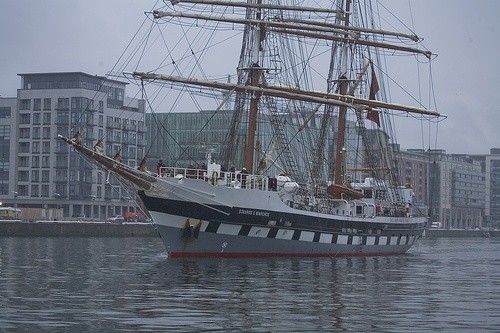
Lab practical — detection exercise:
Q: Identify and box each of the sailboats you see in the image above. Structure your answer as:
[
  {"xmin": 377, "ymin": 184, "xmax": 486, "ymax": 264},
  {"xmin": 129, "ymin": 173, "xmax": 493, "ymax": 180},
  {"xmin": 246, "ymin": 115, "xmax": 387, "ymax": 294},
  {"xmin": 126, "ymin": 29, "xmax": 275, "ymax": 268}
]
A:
[{"xmin": 56, "ymin": 0, "xmax": 448, "ymax": 260}]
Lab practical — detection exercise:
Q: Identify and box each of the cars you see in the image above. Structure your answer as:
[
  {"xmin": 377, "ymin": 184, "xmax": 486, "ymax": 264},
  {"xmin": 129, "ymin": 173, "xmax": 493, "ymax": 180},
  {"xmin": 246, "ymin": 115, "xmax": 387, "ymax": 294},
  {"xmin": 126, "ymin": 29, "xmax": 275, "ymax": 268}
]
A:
[{"xmin": 107, "ymin": 214, "xmax": 124, "ymax": 222}]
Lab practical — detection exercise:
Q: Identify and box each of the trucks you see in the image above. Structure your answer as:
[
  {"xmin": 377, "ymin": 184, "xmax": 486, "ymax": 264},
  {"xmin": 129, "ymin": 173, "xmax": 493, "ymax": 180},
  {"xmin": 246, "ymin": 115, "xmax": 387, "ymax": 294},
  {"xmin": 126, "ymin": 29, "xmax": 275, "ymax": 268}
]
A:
[{"xmin": 431, "ymin": 222, "xmax": 442, "ymax": 229}]
